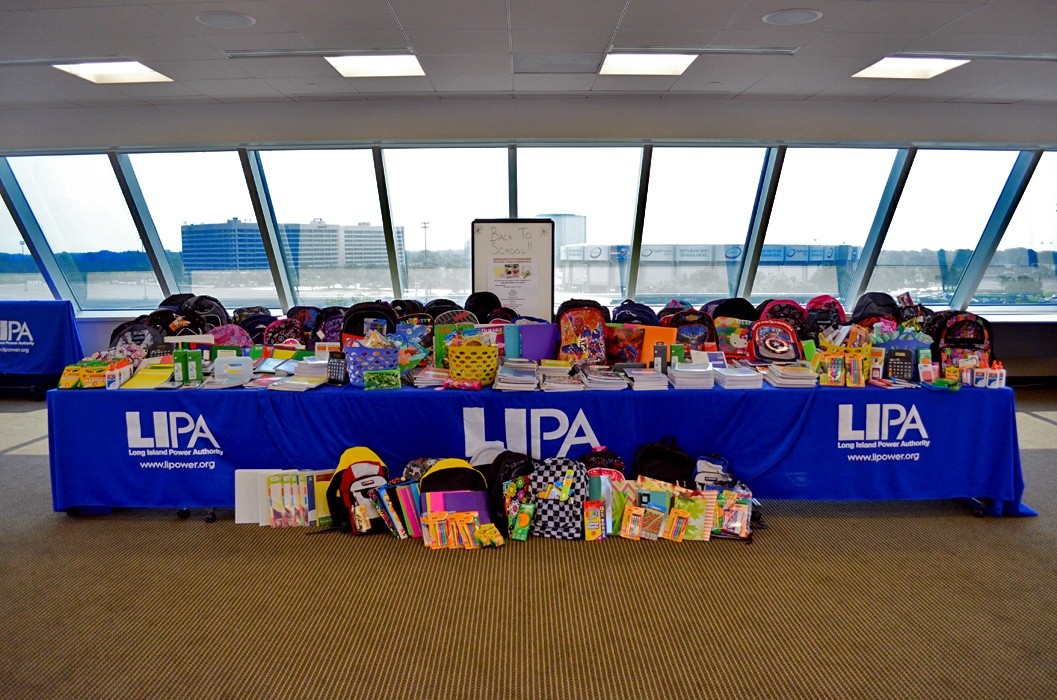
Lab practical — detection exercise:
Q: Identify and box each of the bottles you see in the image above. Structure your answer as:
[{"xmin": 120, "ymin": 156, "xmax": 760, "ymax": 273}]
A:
[
  {"xmin": 187, "ymin": 343, "xmax": 202, "ymax": 381},
  {"xmin": 998, "ymin": 361, "xmax": 1006, "ymax": 388},
  {"xmin": 174, "ymin": 343, "xmax": 190, "ymax": 383},
  {"xmin": 986, "ymin": 360, "xmax": 999, "ymax": 389},
  {"xmin": 106, "ymin": 358, "xmax": 134, "ymax": 389},
  {"xmin": 973, "ymin": 361, "xmax": 986, "ymax": 388},
  {"xmin": 984, "ymin": 360, "xmax": 990, "ymax": 387}
]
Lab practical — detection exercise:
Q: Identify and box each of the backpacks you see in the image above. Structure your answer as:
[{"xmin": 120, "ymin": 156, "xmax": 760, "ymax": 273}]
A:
[
  {"xmin": 659, "ymin": 299, "xmax": 719, "ymax": 360},
  {"xmin": 900, "ymin": 304, "xmax": 935, "ymax": 333},
  {"xmin": 755, "ymin": 300, "xmax": 807, "ymax": 343},
  {"xmin": 693, "ymin": 455, "xmax": 751, "ymax": 539},
  {"xmin": 578, "ymin": 447, "xmax": 624, "ymax": 474},
  {"xmin": 488, "ymin": 452, "xmax": 544, "ymax": 538},
  {"xmin": 555, "ymin": 298, "xmax": 611, "ymax": 365},
  {"xmin": 404, "ymin": 456, "xmax": 439, "ymax": 481},
  {"xmin": 749, "ymin": 320, "xmax": 804, "ymax": 369},
  {"xmin": 926, "ymin": 310, "xmax": 991, "ymax": 366},
  {"xmin": 612, "ymin": 298, "xmax": 659, "ymax": 325},
  {"xmin": 419, "ymin": 458, "xmax": 488, "ymax": 492},
  {"xmin": 109, "ymin": 292, "xmax": 544, "ymax": 360},
  {"xmin": 526, "ymin": 456, "xmax": 588, "ymax": 539},
  {"xmin": 632, "ymin": 437, "xmax": 695, "ymax": 489},
  {"xmin": 588, "ymin": 468, "xmax": 631, "ymax": 536},
  {"xmin": 804, "ymin": 294, "xmax": 848, "ymax": 347},
  {"xmin": 326, "ymin": 447, "xmax": 392, "ymax": 535},
  {"xmin": 851, "ymin": 292, "xmax": 900, "ymax": 331},
  {"xmin": 698, "ymin": 298, "xmax": 759, "ymax": 362}
]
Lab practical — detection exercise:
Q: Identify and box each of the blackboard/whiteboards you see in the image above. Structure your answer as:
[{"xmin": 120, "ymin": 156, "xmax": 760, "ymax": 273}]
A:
[{"xmin": 471, "ymin": 218, "xmax": 554, "ymax": 324}]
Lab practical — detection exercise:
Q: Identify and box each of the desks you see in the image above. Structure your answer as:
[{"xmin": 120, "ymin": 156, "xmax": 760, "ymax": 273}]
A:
[{"xmin": 47, "ymin": 383, "xmax": 1038, "ymax": 522}]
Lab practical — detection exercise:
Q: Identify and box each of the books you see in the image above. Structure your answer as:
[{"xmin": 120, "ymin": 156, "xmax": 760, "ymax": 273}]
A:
[
  {"xmin": 492, "ymin": 357, "xmax": 584, "ymax": 392},
  {"xmin": 434, "ymin": 325, "xmax": 557, "ymax": 367},
  {"xmin": 637, "ymin": 490, "xmax": 669, "ymax": 537},
  {"xmin": 121, "ymin": 357, "xmax": 326, "ymax": 391},
  {"xmin": 414, "ymin": 367, "xmax": 449, "ymax": 385},
  {"xmin": 615, "ymin": 363, "xmax": 668, "ymax": 390},
  {"xmin": 579, "ymin": 370, "xmax": 629, "ymax": 390},
  {"xmin": 667, "ymin": 362, "xmax": 714, "ymax": 389},
  {"xmin": 368, "ymin": 480, "xmax": 487, "ymax": 547},
  {"xmin": 733, "ymin": 358, "xmax": 818, "ymax": 387},
  {"xmin": 713, "ymin": 367, "xmax": 763, "ymax": 388}
]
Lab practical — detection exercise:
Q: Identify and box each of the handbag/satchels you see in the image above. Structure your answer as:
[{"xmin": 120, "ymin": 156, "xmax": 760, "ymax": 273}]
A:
[
  {"xmin": 344, "ymin": 337, "xmax": 401, "ymax": 386},
  {"xmin": 448, "ymin": 338, "xmax": 500, "ymax": 386},
  {"xmin": 872, "ymin": 332, "xmax": 931, "ymax": 384}
]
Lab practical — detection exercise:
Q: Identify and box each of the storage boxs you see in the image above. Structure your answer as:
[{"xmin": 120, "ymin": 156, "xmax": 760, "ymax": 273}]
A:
[
  {"xmin": 215, "ymin": 356, "xmax": 254, "ymax": 380},
  {"xmin": 605, "ymin": 324, "xmax": 677, "ymax": 367}
]
